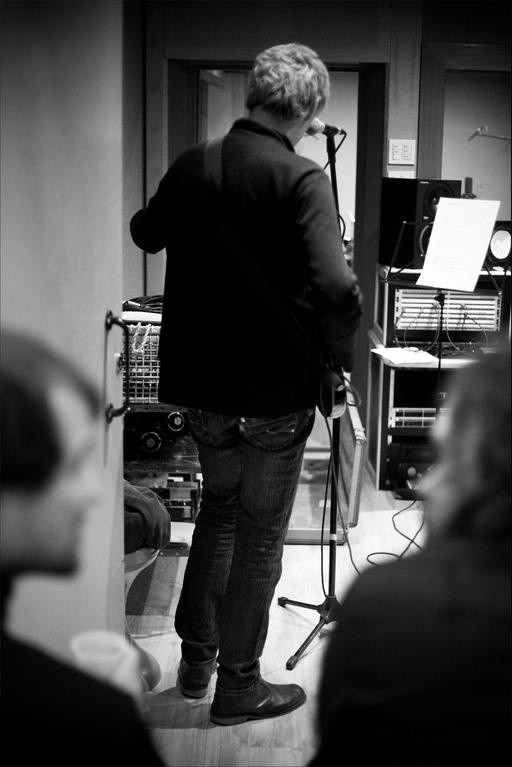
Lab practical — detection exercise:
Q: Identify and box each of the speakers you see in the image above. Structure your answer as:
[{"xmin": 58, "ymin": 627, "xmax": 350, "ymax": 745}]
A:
[{"xmin": 378, "ymin": 176, "xmax": 462, "ymax": 269}]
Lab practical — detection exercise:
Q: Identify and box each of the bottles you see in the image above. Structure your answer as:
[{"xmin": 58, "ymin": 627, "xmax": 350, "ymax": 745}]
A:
[{"xmin": 462, "ymin": 175, "xmax": 475, "ymax": 198}]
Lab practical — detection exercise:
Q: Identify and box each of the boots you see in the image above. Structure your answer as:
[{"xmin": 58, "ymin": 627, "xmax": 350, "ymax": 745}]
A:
[
  {"xmin": 176, "ymin": 640, "xmax": 218, "ymax": 700},
  {"xmin": 210, "ymin": 663, "xmax": 306, "ymax": 726}
]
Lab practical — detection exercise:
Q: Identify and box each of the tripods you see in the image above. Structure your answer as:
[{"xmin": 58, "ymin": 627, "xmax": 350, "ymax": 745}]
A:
[{"xmin": 278, "ymin": 139, "xmax": 344, "ymax": 670}]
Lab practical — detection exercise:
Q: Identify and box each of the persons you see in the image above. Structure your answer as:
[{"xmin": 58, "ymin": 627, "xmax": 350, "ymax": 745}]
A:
[
  {"xmin": 296, "ymin": 345, "xmax": 509, "ymax": 764},
  {"xmin": 0, "ymin": 331, "xmax": 164, "ymax": 767},
  {"xmin": 128, "ymin": 41, "xmax": 367, "ymax": 727}
]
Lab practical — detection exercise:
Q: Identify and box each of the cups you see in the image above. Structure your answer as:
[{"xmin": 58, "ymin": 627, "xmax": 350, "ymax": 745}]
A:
[{"xmin": 69, "ymin": 630, "xmax": 130, "ymax": 680}]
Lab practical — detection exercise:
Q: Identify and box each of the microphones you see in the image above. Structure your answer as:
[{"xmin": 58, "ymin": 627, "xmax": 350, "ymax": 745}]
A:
[
  {"xmin": 305, "ymin": 117, "xmax": 345, "ymax": 135},
  {"xmin": 467, "ymin": 126, "xmax": 485, "ymax": 142}
]
